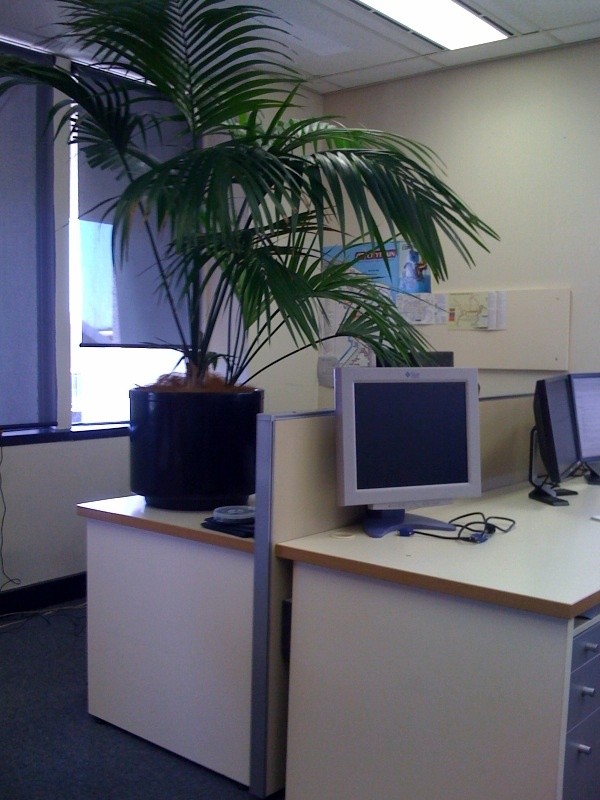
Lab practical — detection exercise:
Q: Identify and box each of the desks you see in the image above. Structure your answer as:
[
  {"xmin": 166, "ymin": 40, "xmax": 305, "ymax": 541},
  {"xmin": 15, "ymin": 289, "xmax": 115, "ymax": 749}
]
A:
[
  {"xmin": 75, "ymin": 493, "xmax": 255, "ymax": 787},
  {"xmin": 275, "ymin": 470, "xmax": 600, "ymax": 800}
]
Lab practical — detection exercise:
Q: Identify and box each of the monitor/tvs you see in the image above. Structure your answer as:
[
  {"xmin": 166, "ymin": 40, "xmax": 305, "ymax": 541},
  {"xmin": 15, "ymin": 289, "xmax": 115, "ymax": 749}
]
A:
[
  {"xmin": 334, "ymin": 365, "xmax": 484, "ymax": 538},
  {"xmin": 526, "ymin": 372, "xmax": 600, "ymax": 507}
]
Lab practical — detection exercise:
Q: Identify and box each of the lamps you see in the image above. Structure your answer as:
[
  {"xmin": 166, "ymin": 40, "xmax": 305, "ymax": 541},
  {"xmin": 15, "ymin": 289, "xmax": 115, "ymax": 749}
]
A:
[{"xmin": 316, "ymin": 334, "xmax": 371, "ymax": 389}]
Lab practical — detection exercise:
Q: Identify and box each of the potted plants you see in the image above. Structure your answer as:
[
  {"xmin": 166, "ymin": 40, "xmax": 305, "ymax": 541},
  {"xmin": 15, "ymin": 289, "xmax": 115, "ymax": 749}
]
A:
[{"xmin": 0, "ymin": 0, "xmax": 498, "ymax": 530}]
